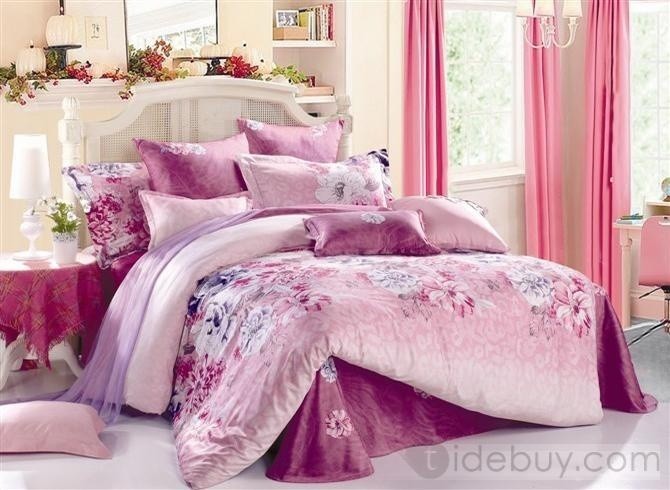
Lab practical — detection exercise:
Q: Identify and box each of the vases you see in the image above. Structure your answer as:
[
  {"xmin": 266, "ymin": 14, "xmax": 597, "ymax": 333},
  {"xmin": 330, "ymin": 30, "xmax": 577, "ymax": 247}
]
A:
[{"xmin": 52, "ymin": 232, "xmax": 80, "ymax": 265}]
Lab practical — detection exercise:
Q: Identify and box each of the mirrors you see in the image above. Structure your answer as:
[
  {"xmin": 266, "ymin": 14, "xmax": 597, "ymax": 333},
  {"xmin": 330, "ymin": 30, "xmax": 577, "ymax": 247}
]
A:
[{"xmin": 125, "ymin": 0, "xmax": 218, "ymax": 69}]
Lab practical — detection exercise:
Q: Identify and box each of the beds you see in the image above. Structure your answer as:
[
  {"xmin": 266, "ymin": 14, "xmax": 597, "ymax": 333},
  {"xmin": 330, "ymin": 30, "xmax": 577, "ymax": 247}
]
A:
[{"xmin": 56, "ymin": 74, "xmax": 659, "ymax": 489}]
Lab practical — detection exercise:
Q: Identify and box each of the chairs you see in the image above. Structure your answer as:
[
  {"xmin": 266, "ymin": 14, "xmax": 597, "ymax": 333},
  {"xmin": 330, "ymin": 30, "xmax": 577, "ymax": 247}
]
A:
[{"xmin": 625, "ymin": 215, "xmax": 669, "ymax": 351}]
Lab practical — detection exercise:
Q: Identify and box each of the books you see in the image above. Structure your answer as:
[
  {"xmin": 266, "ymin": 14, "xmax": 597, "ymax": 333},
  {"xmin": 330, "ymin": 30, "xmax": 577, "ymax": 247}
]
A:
[{"xmin": 299, "ymin": 4, "xmax": 328, "ymax": 40}]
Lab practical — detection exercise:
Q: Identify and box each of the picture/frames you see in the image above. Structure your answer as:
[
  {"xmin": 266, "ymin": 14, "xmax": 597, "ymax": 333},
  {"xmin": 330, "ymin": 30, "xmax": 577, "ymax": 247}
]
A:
[
  {"xmin": 58, "ymin": 0, "xmax": 129, "ymax": 76},
  {"xmin": 275, "ymin": 9, "xmax": 300, "ymax": 28}
]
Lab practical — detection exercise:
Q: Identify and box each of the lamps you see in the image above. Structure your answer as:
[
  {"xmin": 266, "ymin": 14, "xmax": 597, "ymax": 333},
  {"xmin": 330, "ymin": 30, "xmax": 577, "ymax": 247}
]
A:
[
  {"xmin": 8, "ymin": 133, "xmax": 55, "ymax": 262},
  {"xmin": 44, "ymin": 11, "xmax": 85, "ymax": 70},
  {"xmin": 14, "ymin": 38, "xmax": 47, "ymax": 80},
  {"xmin": 513, "ymin": 0, "xmax": 583, "ymax": 51},
  {"xmin": 171, "ymin": 40, "xmax": 278, "ymax": 77}
]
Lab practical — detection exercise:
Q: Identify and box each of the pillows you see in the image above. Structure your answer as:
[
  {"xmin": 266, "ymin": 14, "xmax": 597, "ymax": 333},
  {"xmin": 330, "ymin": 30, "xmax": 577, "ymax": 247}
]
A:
[
  {"xmin": 0, "ymin": 400, "xmax": 116, "ymax": 464},
  {"xmin": 62, "ymin": 112, "xmax": 513, "ymax": 261}
]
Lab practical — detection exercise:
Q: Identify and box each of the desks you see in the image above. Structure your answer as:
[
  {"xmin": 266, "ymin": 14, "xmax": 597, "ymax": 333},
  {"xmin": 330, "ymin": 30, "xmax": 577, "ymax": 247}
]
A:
[
  {"xmin": 612, "ymin": 200, "xmax": 670, "ymax": 330},
  {"xmin": 0, "ymin": 252, "xmax": 102, "ymax": 395}
]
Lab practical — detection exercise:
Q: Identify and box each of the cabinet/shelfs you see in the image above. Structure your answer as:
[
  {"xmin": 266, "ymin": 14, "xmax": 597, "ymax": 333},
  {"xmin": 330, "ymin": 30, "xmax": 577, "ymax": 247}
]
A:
[{"xmin": 271, "ymin": 40, "xmax": 337, "ymax": 104}]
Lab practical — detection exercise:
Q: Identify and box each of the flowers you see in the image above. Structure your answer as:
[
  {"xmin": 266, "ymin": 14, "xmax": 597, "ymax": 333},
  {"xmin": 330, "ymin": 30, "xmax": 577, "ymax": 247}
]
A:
[{"xmin": 22, "ymin": 193, "xmax": 83, "ymax": 242}]
